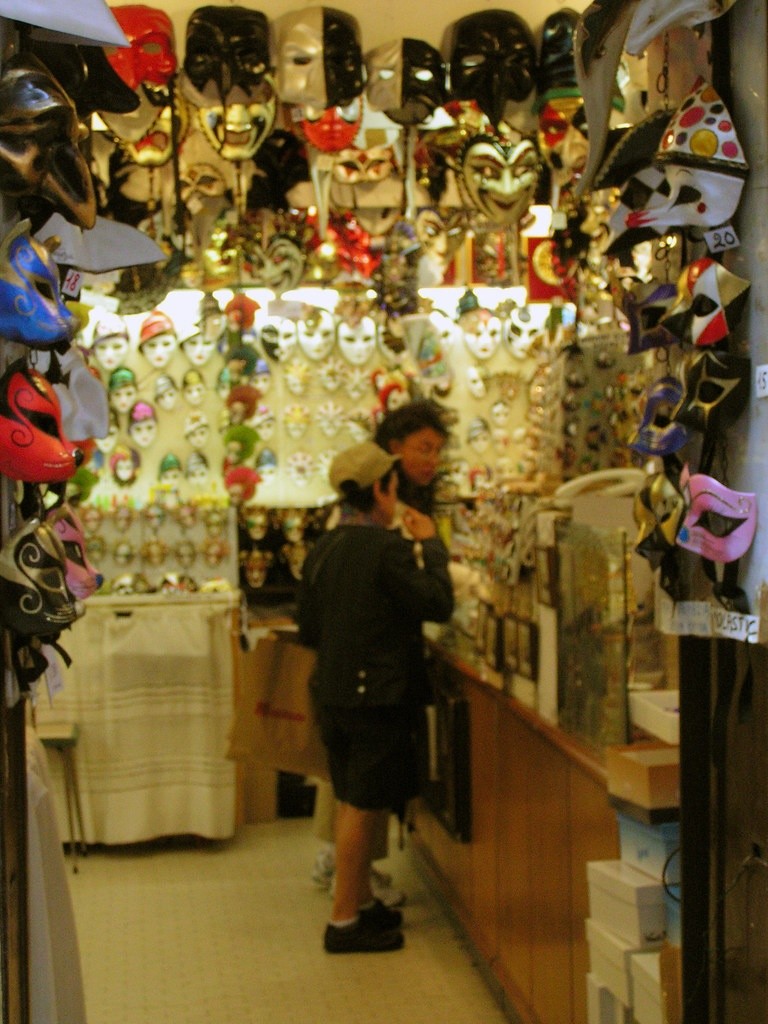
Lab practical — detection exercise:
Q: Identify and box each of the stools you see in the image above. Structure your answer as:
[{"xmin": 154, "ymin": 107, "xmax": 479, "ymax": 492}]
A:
[{"xmin": 37, "ymin": 721, "xmax": 91, "ymax": 874}]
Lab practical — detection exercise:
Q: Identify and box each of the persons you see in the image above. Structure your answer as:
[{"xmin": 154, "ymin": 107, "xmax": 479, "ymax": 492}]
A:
[
  {"xmin": 311, "ymin": 403, "xmax": 447, "ymax": 905},
  {"xmin": 297, "ymin": 441, "xmax": 458, "ymax": 953}
]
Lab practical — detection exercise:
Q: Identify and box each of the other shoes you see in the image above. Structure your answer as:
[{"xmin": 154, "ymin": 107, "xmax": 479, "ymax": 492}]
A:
[
  {"xmin": 332, "ymin": 899, "xmax": 407, "ymax": 928},
  {"xmin": 325, "ymin": 918, "xmax": 406, "ymax": 954},
  {"xmin": 328, "ymin": 874, "xmax": 409, "ymax": 906},
  {"xmin": 308, "ymin": 848, "xmax": 393, "ymax": 889}
]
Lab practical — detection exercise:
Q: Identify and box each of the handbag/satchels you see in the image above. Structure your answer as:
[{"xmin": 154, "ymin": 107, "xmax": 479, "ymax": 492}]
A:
[{"xmin": 225, "ymin": 630, "xmax": 335, "ymax": 786}]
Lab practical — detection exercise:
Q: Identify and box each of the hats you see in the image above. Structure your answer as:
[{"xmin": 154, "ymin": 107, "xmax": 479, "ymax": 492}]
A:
[{"xmin": 329, "ymin": 441, "xmax": 401, "ymax": 494}]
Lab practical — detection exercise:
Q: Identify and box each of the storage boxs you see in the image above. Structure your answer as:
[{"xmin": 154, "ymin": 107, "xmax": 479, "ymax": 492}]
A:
[{"xmin": 583, "ymin": 688, "xmax": 680, "ymax": 1022}]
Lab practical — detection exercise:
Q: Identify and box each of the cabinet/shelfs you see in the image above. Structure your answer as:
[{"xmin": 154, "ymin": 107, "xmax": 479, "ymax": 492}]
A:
[{"xmin": 415, "ymin": 675, "xmax": 672, "ymax": 1024}]
